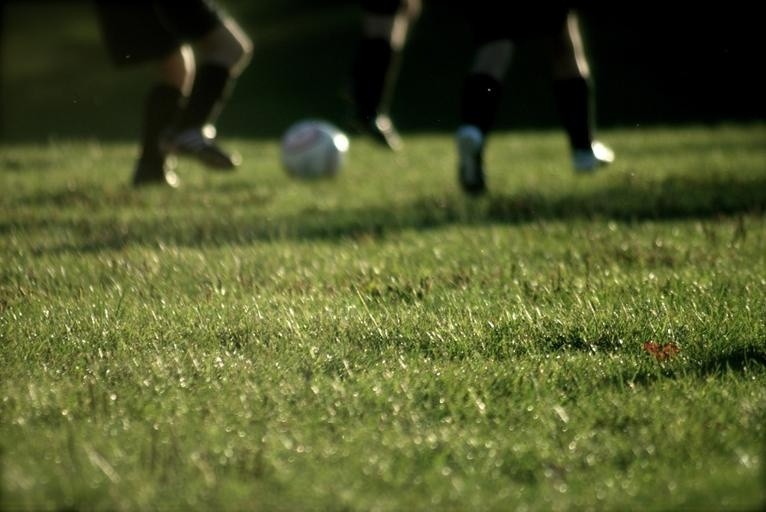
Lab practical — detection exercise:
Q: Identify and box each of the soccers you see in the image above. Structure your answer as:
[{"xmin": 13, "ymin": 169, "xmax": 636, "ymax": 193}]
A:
[{"xmin": 283, "ymin": 123, "xmax": 345, "ymax": 181}]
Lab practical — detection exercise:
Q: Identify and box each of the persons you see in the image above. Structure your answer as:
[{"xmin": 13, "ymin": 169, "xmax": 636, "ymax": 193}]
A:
[
  {"xmin": 353, "ymin": 1, "xmax": 423, "ymax": 151},
  {"xmin": 452, "ymin": 3, "xmax": 617, "ymax": 196},
  {"xmin": 91, "ymin": 0, "xmax": 253, "ymax": 190}
]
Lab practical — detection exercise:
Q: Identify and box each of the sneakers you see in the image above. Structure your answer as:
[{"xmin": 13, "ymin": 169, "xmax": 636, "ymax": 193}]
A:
[
  {"xmin": 457, "ymin": 126, "xmax": 488, "ymax": 197},
  {"xmin": 156, "ymin": 122, "xmax": 239, "ymax": 170},
  {"xmin": 136, "ymin": 152, "xmax": 179, "ymax": 188},
  {"xmin": 573, "ymin": 142, "xmax": 616, "ymax": 169}
]
